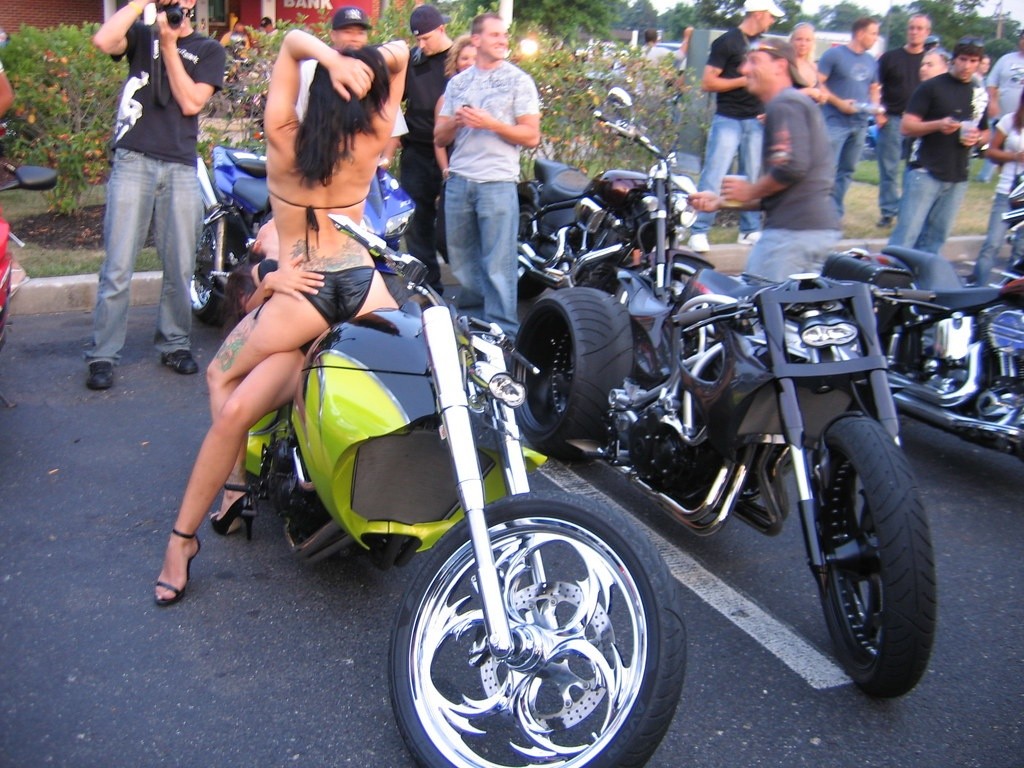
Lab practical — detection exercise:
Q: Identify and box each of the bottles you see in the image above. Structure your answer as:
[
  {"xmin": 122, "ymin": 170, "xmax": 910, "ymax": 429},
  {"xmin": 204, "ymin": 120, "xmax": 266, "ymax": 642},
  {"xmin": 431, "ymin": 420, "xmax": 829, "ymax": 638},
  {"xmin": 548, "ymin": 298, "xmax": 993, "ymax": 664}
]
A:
[{"xmin": 853, "ymin": 102, "xmax": 884, "ymax": 114}]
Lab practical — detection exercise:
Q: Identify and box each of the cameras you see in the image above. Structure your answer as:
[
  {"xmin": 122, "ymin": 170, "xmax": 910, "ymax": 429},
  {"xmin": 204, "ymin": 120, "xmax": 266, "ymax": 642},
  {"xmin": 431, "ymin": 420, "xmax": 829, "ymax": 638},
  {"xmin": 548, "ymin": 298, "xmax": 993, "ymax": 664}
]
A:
[{"xmin": 156, "ymin": 0, "xmax": 186, "ymax": 29}]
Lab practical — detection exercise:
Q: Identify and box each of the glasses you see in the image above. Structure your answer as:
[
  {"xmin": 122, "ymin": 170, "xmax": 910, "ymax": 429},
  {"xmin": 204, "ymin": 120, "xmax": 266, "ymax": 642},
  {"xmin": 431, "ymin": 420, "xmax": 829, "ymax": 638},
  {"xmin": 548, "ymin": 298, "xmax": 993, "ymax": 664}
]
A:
[
  {"xmin": 747, "ymin": 43, "xmax": 778, "ymax": 51},
  {"xmin": 957, "ymin": 38, "xmax": 985, "ymax": 47}
]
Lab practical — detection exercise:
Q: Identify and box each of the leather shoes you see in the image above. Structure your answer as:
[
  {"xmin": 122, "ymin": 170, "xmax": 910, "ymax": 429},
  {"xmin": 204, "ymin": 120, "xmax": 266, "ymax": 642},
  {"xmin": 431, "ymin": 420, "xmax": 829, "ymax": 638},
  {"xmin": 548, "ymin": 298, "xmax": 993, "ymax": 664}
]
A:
[
  {"xmin": 162, "ymin": 349, "xmax": 199, "ymax": 374},
  {"xmin": 87, "ymin": 361, "xmax": 113, "ymax": 389}
]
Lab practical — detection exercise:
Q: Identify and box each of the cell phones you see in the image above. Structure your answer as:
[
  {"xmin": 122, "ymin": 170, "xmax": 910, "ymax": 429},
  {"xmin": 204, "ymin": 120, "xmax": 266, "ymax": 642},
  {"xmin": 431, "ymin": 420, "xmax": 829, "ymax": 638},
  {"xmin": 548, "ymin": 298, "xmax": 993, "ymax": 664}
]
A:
[{"xmin": 462, "ymin": 104, "xmax": 470, "ymax": 108}]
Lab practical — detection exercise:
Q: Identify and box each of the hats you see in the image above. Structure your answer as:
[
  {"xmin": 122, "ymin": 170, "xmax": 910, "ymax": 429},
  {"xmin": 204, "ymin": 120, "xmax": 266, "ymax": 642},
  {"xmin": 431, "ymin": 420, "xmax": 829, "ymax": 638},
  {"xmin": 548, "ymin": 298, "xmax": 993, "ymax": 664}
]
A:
[
  {"xmin": 410, "ymin": 5, "xmax": 452, "ymax": 36},
  {"xmin": 332, "ymin": 7, "xmax": 373, "ymax": 29},
  {"xmin": 743, "ymin": 0, "xmax": 786, "ymax": 18},
  {"xmin": 749, "ymin": 36, "xmax": 808, "ymax": 89},
  {"xmin": 260, "ymin": 17, "xmax": 271, "ymax": 27}
]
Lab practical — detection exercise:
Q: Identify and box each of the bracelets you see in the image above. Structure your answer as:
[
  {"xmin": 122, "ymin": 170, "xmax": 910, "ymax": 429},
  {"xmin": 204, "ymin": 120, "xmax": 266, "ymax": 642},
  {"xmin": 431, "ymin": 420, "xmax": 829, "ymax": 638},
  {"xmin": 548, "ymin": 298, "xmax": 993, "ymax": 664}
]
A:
[
  {"xmin": 977, "ymin": 134, "xmax": 983, "ymax": 143},
  {"xmin": 129, "ymin": 1, "xmax": 142, "ymax": 15}
]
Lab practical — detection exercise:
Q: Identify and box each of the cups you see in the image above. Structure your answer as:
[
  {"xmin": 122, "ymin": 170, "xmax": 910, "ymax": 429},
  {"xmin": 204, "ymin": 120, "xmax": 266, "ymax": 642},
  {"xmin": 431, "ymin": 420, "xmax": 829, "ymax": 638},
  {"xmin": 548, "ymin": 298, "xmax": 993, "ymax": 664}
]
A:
[
  {"xmin": 959, "ymin": 121, "xmax": 976, "ymax": 141},
  {"xmin": 722, "ymin": 175, "xmax": 747, "ymax": 209}
]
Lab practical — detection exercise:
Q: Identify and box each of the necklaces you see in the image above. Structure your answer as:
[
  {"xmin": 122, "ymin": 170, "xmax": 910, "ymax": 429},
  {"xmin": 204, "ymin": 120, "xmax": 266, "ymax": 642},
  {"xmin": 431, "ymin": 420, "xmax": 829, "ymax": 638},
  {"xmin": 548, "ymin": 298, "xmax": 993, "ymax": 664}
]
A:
[{"xmin": 741, "ymin": 29, "xmax": 759, "ymax": 46}]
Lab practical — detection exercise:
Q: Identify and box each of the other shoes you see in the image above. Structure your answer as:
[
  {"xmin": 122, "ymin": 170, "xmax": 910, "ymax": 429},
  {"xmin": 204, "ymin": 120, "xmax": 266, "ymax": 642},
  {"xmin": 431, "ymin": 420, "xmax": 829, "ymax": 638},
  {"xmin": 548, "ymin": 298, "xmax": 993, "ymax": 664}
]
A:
[{"xmin": 876, "ymin": 216, "xmax": 892, "ymax": 228}]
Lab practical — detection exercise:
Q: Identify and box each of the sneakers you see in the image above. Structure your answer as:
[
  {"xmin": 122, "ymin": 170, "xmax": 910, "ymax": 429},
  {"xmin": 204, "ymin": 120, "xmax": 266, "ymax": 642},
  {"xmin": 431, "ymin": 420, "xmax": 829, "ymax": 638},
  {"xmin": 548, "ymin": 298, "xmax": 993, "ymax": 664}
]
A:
[
  {"xmin": 687, "ymin": 233, "xmax": 710, "ymax": 251},
  {"xmin": 737, "ymin": 231, "xmax": 761, "ymax": 244}
]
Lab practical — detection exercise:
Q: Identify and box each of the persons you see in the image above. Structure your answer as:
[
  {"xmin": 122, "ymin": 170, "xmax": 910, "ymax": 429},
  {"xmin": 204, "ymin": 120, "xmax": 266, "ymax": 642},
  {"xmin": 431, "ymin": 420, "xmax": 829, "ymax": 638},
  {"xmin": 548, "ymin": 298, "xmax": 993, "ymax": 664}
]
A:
[
  {"xmin": 645, "ymin": 1, "xmax": 1024, "ymax": 286},
  {"xmin": 295, "ymin": 7, "xmax": 408, "ymax": 171},
  {"xmin": 0, "ymin": 61, "xmax": 13, "ymax": 118},
  {"xmin": 207, "ymin": 30, "xmax": 409, "ymax": 542},
  {"xmin": 220, "ymin": 21, "xmax": 250, "ymax": 50},
  {"xmin": 433, "ymin": 14, "xmax": 541, "ymax": 372},
  {"xmin": 85, "ymin": 0, "xmax": 225, "ymax": 389},
  {"xmin": 402, "ymin": 6, "xmax": 478, "ymax": 297},
  {"xmin": 154, "ymin": 217, "xmax": 321, "ymax": 605},
  {"xmin": 258, "ymin": 17, "xmax": 278, "ymax": 37}
]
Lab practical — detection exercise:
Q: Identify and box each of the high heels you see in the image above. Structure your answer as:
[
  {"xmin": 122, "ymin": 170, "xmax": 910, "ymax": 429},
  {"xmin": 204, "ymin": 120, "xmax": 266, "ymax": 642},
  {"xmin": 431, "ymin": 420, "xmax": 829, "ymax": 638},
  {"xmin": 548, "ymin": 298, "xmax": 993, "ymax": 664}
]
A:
[
  {"xmin": 211, "ymin": 484, "xmax": 259, "ymax": 541},
  {"xmin": 154, "ymin": 527, "xmax": 200, "ymax": 606}
]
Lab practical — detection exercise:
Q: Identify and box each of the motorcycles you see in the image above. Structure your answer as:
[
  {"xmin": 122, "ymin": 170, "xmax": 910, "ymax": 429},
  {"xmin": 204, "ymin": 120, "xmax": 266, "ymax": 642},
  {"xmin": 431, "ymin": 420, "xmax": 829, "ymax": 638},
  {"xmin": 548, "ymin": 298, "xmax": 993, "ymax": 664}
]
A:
[
  {"xmin": 0, "ymin": 166, "xmax": 59, "ymax": 408},
  {"xmin": 519, "ymin": 88, "xmax": 716, "ymax": 384},
  {"xmin": 509, "ymin": 255, "xmax": 936, "ymax": 696},
  {"xmin": 244, "ymin": 213, "xmax": 688, "ymax": 768},
  {"xmin": 790, "ymin": 168, "xmax": 1024, "ymax": 458},
  {"xmin": 189, "ymin": 140, "xmax": 417, "ymax": 324}
]
